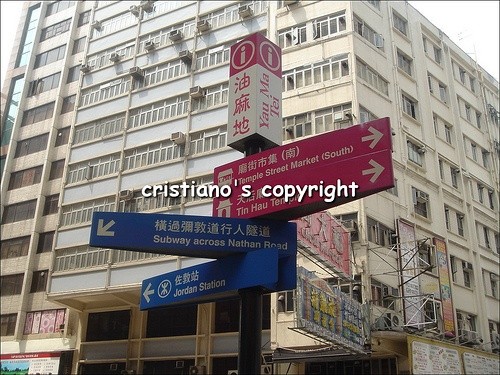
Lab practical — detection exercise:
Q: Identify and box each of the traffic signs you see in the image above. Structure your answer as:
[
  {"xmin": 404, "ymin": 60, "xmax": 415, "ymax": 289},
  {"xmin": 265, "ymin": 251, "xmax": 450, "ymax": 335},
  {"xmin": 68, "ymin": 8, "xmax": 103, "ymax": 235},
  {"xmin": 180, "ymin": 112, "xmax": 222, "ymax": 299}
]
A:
[
  {"xmin": 141, "ymin": 259, "xmax": 297, "ymax": 311},
  {"xmin": 89, "ymin": 211, "xmax": 298, "ymax": 259},
  {"xmin": 212, "ymin": 149, "xmax": 393, "ymax": 222},
  {"xmin": 213, "ymin": 117, "xmax": 393, "ymax": 197}
]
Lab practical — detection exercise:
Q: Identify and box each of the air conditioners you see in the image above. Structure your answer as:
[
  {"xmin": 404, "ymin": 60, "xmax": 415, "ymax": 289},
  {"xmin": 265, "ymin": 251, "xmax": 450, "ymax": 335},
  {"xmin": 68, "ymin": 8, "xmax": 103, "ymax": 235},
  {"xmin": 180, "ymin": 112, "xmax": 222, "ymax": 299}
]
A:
[
  {"xmin": 171, "ymin": 131, "xmax": 185, "ymax": 144},
  {"xmin": 119, "ymin": 189, "xmax": 132, "ymax": 200},
  {"xmin": 109, "ymin": 53, "xmax": 120, "ymax": 61},
  {"xmin": 196, "ymin": 19, "xmax": 211, "ymax": 31},
  {"xmin": 178, "ymin": 49, "xmax": 192, "ymax": 63},
  {"xmin": 169, "ymin": 30, "xmax": 183, "ymax": 40},
  {"xmin": 384, "ymin": 313, "xmax": 402, "ymax": 332},
  {"xmin": 129, "ymin": 5, "xmax": 138, "ymax": 15},
  {"xmin": 140, "ymin": 0, "xmax": 152, "ymax": 9},
  {"xmin": 145, "ymin": 41, "xmax": 154, "ymax": 50},
  {"xmin": 129, "ymin": 66, "xmax": 143, "ymax": 79},
  {"xmin": 238, "ymin": 5, "xmax": 252, "ymax": 19},
  {"xmin": 91, "ymin": 19, "xmax": 99, "ymax": 28},
  {"xmin": 80, "ymin": 64, "xmax": 90, "ymax": 72},
  {"xmin": 190, "ymin": 85, "xmax": 203, "ymax": 98}
]
